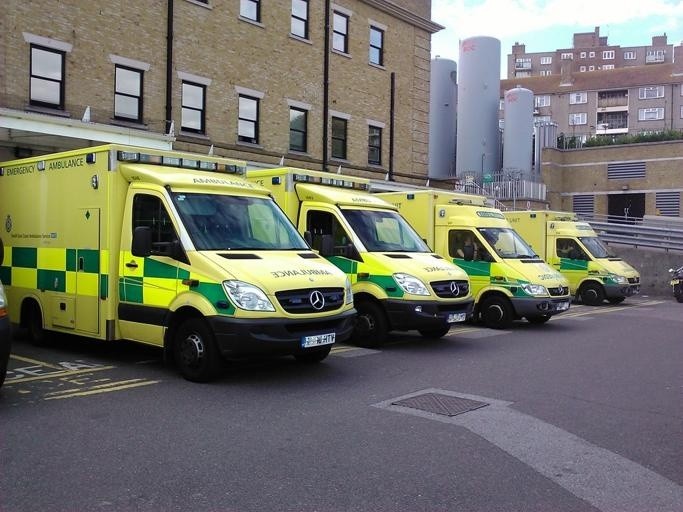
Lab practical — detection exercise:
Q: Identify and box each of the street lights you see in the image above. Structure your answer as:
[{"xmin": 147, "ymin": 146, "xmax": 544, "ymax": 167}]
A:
[{"xmin": 480, "ymin": 153, "xmax": 487, "ymax": 180}]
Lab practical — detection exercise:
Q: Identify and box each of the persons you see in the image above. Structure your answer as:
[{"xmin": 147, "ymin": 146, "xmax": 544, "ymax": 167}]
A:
[
  {"xmin": 478, "ymin": 232, "xmax": 499, "ymax": 260},
  {"xmin": 455, "ymin": 235, "xmax": 478, "ymax": 260}
]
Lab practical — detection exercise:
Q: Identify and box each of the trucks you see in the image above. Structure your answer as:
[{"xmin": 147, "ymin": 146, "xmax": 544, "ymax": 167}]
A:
[
  {"xmin": 0, "ymin": 237, "xmax": 10, "ymax": 380},
  {"xmin": 500, "ymin": 209, "xmax": 641, "ymax": 306},
  {"xmin": 244, "ymin": 167, "xmax": 477, "ymax": 347},
  {"xmin": 369, "ymin": 187, "xmax": 574, "ymax": 333},
  {"xmin": 0, "ymin": 144, "xmax": 358, "ymax": 385}
]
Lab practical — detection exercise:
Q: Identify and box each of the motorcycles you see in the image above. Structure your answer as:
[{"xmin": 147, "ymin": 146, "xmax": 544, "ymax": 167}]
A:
[{"xmin": 668, "ymin": 266, "xmax": 682, "ymax": 303}]
